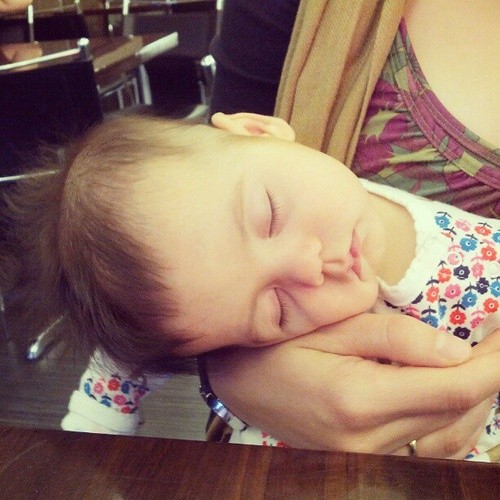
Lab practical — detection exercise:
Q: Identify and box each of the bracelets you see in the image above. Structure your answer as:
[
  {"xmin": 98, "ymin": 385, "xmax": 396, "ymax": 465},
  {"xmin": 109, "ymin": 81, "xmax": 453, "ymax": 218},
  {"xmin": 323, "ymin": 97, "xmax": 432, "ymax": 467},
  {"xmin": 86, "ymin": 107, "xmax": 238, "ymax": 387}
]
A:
[{"xmin": 193, "ymin": 361, "xmax": 247, "ymax": 435}]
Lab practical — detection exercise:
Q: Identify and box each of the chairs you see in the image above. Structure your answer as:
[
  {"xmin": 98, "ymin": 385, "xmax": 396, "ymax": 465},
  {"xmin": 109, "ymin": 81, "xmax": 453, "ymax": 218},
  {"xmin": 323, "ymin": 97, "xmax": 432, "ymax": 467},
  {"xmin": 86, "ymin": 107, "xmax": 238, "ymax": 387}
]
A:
[
  {"xmin": 123, "ymin": 0, "xmax": 227, "ymax": 121},
  {"xmin": 0, "ymin": 36, "xmax": 112, "ymax": 355}
]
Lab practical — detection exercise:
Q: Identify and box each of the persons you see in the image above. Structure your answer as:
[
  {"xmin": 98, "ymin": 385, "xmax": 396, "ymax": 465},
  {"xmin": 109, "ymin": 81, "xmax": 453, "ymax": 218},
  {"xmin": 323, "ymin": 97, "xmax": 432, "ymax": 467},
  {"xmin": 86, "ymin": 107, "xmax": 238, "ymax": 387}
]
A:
[
  {"xmin": 204, "ymin": 0, "xmax": 500, "ymax": 465},
  {"xmin": 4, "ymin": 106, "xmax": 500, "ymax": 466}
]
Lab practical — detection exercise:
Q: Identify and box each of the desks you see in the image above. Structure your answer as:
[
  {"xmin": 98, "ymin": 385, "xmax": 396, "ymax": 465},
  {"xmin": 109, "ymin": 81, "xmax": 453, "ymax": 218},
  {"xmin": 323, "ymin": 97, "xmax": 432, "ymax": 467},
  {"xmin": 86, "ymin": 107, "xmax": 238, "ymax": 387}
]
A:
[
  {"xmin": 0, "ymin": 32, "xmax": 145, "ymax": 131},
  {"xmin": 0, "ymin": 421, "xmax": 500, "ymax": 500}
]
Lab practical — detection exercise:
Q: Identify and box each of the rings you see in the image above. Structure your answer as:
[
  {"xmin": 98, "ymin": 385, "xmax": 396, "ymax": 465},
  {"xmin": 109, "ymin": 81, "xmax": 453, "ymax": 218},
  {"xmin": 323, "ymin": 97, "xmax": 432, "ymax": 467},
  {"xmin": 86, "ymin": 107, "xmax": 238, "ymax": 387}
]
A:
[{"xmin": 408, "ymin": 440, "xmax": 422, "ymax": 461}]
